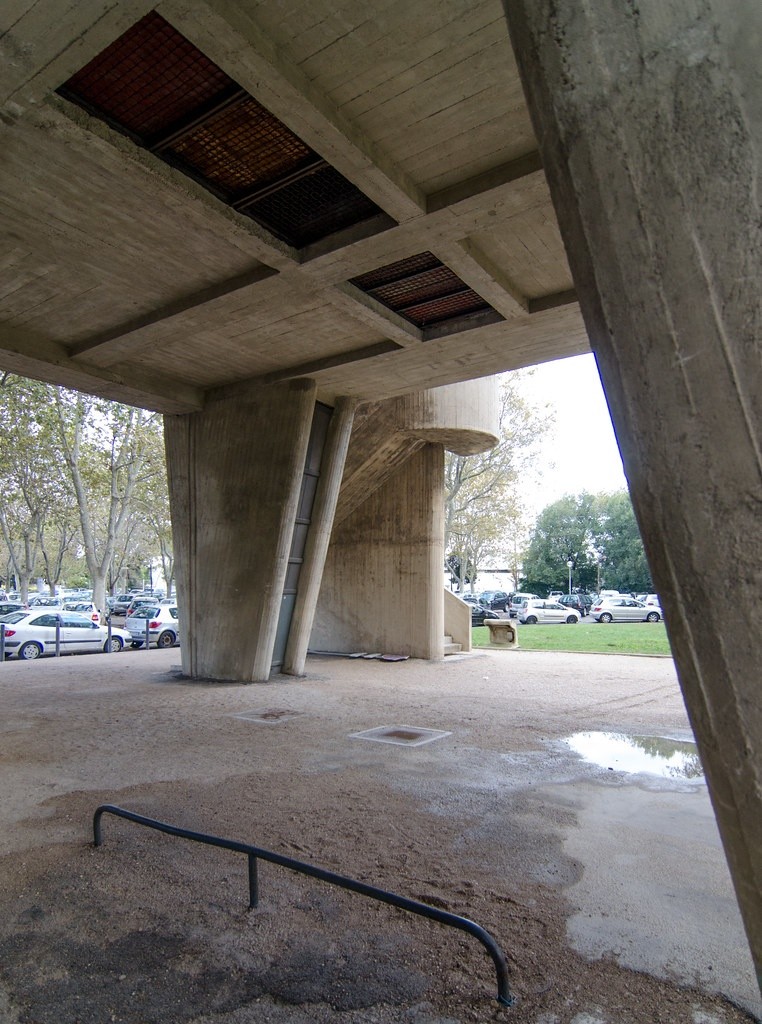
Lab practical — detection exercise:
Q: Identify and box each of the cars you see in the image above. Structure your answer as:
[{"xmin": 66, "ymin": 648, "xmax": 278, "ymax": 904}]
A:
[
  {"xmin": 0, "ymin": 586, "xmax": 179, "ymax": 660},
  {"xmin": 458, "ymin": 591, "xmax": 661, "ymax": 626}
]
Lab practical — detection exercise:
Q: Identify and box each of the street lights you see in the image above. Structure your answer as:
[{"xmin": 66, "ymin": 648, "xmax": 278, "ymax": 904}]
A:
[{"xmin": 566, "ymin": 561, "xmax": 574, "ymax": 595}]
